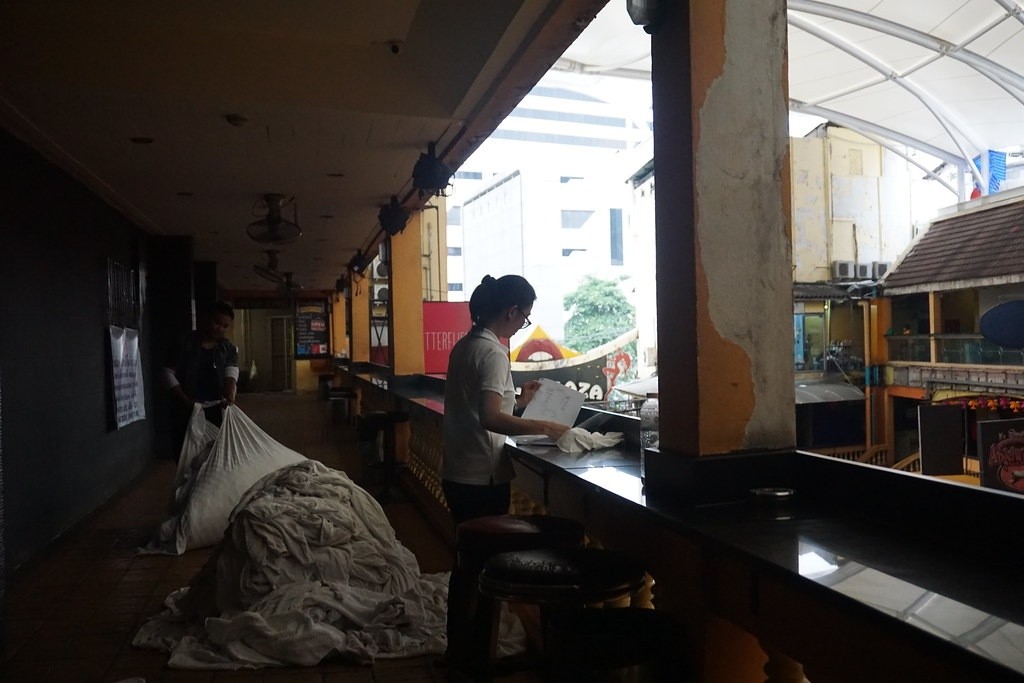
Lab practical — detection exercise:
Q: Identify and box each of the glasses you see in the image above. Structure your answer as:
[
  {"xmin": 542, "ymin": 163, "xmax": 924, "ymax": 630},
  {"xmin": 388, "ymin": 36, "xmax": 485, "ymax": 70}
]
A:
[{"xmin": 517, "ymin": 306, "xmax": 531, "ymax": 330}]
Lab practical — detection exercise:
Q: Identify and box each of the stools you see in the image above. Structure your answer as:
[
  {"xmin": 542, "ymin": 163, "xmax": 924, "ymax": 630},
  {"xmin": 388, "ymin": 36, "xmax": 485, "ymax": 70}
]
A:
[
  {"xmin": 325, "ymin": 388, "xmax": 358, "ymax": 428},
  {"xmin": 318, "ymin": 373, "xmax": 335, "ymax": 402},
  {"xmin": 444, "ymin": 512, "xmax": 647, "ymax": 683},
  {"xmin": 367, "ymin": 408, "xmax": 410, "ymax": 475}
]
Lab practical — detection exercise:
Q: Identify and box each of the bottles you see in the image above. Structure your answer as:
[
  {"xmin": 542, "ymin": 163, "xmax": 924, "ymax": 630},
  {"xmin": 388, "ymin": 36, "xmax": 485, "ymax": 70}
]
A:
[{"xmin": 640, "ymin": 392, "xmax": 659, "ymax": 478}]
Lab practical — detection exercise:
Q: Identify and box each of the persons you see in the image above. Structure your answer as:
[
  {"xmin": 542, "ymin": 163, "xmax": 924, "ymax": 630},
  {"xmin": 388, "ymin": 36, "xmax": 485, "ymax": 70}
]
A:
[
  {"xmin": 154, "ymin": 304, "xmax": 241, "ymax": 450},
  {"xmin": 442, "ymin": 274, "xmax": 574, "ymax": 675}
]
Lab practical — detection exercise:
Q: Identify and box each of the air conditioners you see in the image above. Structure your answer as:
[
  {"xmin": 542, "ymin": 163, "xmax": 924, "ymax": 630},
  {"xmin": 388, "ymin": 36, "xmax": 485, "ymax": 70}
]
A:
[
  {"xmin": 855, "ymin": 263, "xmax": 873, "ymax": 279},
  {"xmin": 833, "ymin": 260, "xmax": 855, "ymax": 279},
  {"xmin": 372, "ymin": 284, "xmax": 388, "ymax": 300},
  {"xmin": 372, "ymin": 258, "xmax": 388, "ymax": 280},
  {"xmin": 873, "ymin": 261, "xmax": 891, "ymax": 279}
]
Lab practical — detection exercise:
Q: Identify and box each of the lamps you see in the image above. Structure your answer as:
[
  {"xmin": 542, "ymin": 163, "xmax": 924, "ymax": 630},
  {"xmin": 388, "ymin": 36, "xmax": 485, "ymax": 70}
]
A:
[
  {"xmin": 348, "ymin": 248, "xmax": 370, "ymax": 274},
  {"xmin": 377, "ymin": 196, "xmax": 410, "ymax": 236},
  {"xmin": 335, "ymin": 275, "xmax": 348, "ymax": 291},
  {"xmin": 413, "ymin": 142, "xmax": 450, "ymax": 200}
]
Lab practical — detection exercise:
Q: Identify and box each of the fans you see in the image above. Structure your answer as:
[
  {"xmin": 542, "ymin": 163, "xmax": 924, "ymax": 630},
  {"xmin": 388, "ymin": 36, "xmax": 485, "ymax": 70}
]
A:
[
  {"xmin": 254, "ymin": 259, "xmax": 303, "ymax": 290},
  {"xmin": 247, "ymin": 194, "xmax": 304, "ymax": 246}
]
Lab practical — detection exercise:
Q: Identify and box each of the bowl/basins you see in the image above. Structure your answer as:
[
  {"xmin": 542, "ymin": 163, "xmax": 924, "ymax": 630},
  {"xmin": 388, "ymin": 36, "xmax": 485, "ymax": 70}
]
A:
[{"xmin": 749, "ymin": 488, "xmax": 797, "ymax": 520}]
